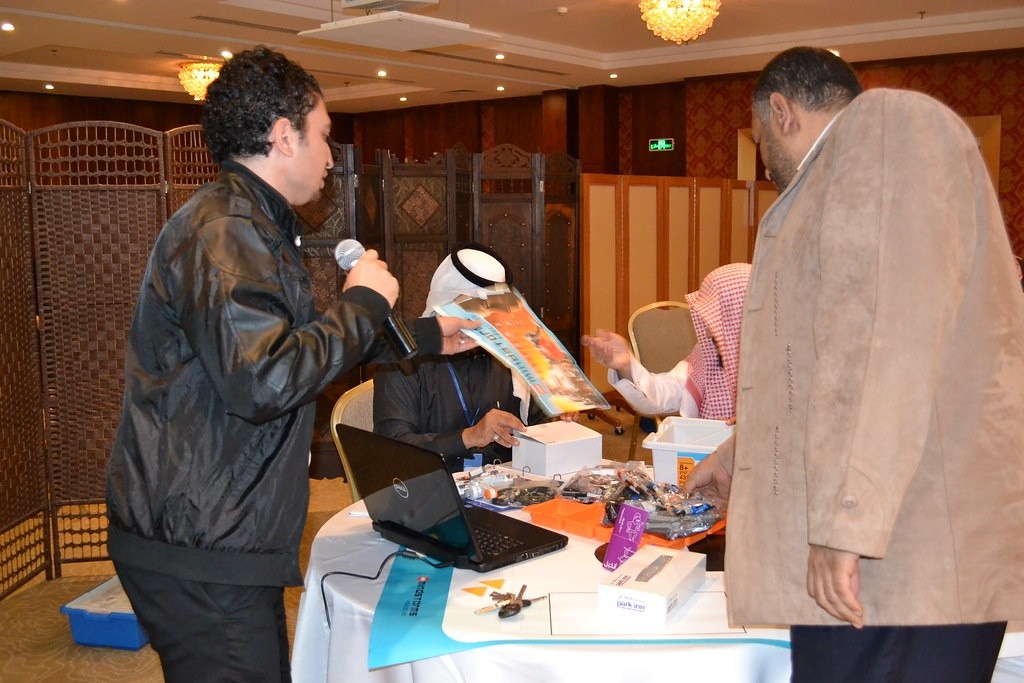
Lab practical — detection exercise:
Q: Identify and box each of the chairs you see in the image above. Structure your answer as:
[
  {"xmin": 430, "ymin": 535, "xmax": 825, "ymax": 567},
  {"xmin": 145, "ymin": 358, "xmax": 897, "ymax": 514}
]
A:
[
  {"xmin": 578, "ymin": 300, "xmax": 699, "ymax": 462},
  {"xmin": 329, "ymin": 377, "xmax": 374, "ymax": 503}
]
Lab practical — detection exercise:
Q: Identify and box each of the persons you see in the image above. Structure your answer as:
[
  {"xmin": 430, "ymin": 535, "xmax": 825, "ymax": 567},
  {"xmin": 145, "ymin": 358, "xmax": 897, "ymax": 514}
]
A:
[
  {"xmin": 103, "ymin": 46, "xmax": 483, "ymax": 683},
  {"xmin": 580, "ymin": 263, "xmax": 752, "ymax": 425},
  {"xmin": 371, "ymin": 244, "xmax": 581, "ymax": 476},
  {"xmin": 676, "ymin": 46, "xmax": 1024, "ymax": 683}
]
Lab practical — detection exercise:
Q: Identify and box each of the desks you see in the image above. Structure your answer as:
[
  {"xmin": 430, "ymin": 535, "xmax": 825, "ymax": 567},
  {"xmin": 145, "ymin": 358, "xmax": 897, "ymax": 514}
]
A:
[{"xmin": 291, "ymin": 458, "xmax": 794, "ymax": 683}]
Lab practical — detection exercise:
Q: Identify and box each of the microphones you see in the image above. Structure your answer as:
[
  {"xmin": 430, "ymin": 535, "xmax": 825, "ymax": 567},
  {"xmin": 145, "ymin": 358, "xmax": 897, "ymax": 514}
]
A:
[{"xmin": 335, "ymin": 238, "xmax": 418, "ymax": 359}]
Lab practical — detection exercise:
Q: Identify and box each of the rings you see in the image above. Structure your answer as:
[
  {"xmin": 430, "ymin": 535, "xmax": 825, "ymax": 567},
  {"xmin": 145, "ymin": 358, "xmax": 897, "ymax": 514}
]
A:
[
  {"xmin": 460, "ymin": 340, "xmax": 465, "ymax": 344},
  {"xmin": 492, "ymin": 434, "xmax": 500, "ymax": 442}
]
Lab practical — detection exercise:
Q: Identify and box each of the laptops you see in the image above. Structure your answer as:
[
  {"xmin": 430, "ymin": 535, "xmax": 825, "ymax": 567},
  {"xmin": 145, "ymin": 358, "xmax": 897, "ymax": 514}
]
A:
[{"xmin": 335, "ymin": 423, "xmax": 568, "ymax": 573}]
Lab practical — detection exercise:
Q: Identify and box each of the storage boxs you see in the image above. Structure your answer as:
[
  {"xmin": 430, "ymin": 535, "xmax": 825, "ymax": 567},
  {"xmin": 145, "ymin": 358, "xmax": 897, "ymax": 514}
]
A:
[
  {"xmin": 512, "ymin": 420, "xmax": 603, "ymax": 478},
  {"xmin": 641, "ymin": 415, "xmax": 736, "ymax": 489},
  {"xmin": 597, "ymin": 544, "xmax": 708, "ymax": 625},
  {"xmin": 59, "ymin": 574, "xmax": 149, "ymax": 650}
]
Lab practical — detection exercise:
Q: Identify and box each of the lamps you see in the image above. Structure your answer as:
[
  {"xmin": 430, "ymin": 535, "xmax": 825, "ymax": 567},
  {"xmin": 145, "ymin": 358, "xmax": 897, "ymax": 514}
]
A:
[
  {"xmin": 177, "ymin": 61, "xmax": 225, "ymax": 101},
  {"xmin": 637, "ymin": 0, "xmax": 722, "ymax": 45}
]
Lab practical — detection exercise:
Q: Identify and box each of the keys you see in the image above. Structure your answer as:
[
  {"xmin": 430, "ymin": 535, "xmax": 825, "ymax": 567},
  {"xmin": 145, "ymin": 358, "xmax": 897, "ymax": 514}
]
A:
[{"xmin": 473, "ymin": 584, "xmax": 548, "ymax": 619}]
